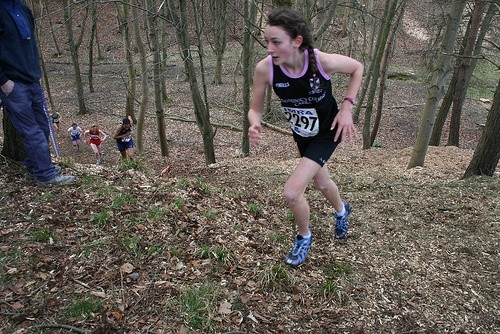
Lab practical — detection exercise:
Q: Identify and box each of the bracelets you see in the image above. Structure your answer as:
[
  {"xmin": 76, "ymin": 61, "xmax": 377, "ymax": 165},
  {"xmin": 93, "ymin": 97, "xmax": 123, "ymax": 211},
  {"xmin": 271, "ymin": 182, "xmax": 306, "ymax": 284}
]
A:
[{"xmin": 343, "ymin": 97, "xmax": 357, "ymax": 105}]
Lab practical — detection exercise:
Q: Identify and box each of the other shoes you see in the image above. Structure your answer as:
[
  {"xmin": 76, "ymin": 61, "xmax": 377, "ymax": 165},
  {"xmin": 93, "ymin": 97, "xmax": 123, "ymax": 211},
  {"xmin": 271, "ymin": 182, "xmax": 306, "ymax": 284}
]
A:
[{"xmin": 95, "ymin": 158, "xmax": 101, "ymax": 165}]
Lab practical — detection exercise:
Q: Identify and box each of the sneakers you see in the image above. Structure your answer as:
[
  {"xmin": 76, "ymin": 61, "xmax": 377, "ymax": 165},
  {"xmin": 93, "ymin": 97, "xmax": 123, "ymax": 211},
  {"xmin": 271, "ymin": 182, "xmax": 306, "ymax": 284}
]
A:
[
  {"xmin": 286, "ymin": 229, "xmax": 314, "ymax": 266},
  {"xmin": 332, "ymin": 201, "xmax": 351, "ymax": 239},
  {"xmin": 37, "ymin": 175, "xmax": 76, "ymax": 187},
  {"xmin": 25, "ymin": 164, "xmax": 61, "ymax": 183}
]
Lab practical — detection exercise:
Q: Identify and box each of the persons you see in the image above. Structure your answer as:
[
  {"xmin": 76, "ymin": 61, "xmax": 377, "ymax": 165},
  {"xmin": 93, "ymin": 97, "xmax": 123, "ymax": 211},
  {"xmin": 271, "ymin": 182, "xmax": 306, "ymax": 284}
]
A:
[
  {"xmin": 66, "ymin": 123, "xmax": 82, "ymax": 151},
  {"xmin": 48, "ymin": 108, "xmax": 62, "ymax": 138},
  {"xmin": 0, "ymin": 0, "xmax": 76, "ymax": 187},
  {"xmin": 112, "ymin": 115, "xmax": 136, "ymax": 163},
  {"xmin": 83, "ymin": 124, "xmax": 107, "ymax": 165},
  {"xmin": 248, "ymin": 6, "xmax": 365, "ymax": 265}
]
